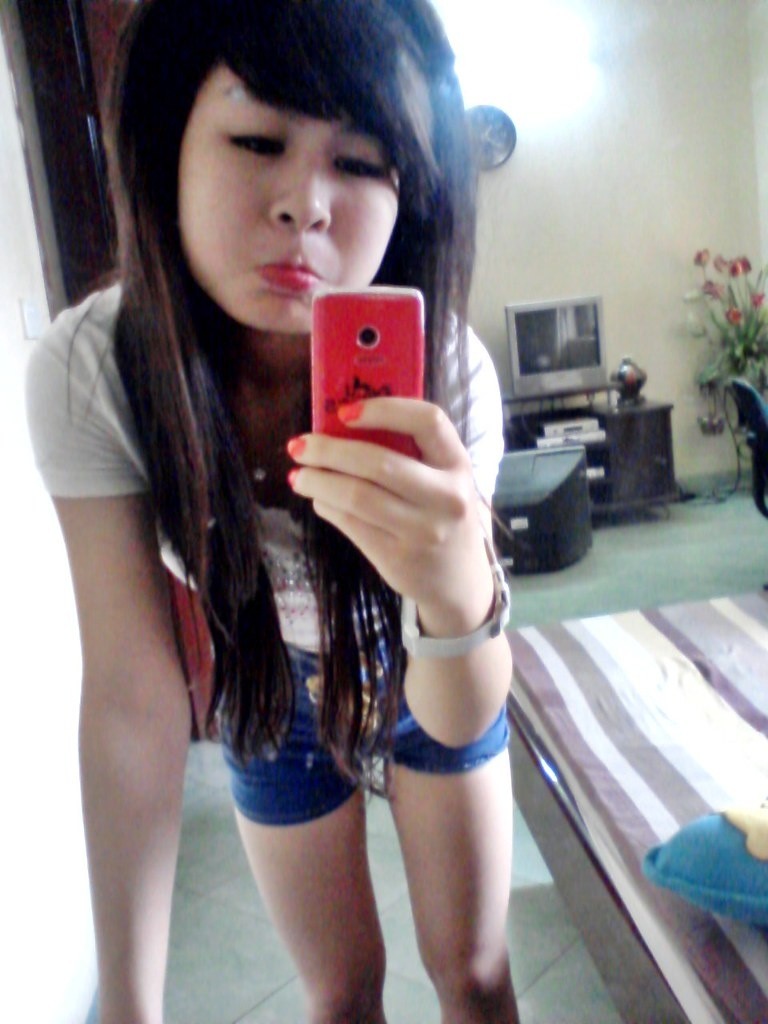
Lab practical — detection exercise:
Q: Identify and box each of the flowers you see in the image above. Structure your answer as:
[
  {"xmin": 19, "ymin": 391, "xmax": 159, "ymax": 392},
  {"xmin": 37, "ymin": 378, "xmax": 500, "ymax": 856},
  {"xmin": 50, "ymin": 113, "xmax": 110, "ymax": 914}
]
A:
[{"xmin": 686, "ymin": 250, "xmax": 768, "ymax": 452}]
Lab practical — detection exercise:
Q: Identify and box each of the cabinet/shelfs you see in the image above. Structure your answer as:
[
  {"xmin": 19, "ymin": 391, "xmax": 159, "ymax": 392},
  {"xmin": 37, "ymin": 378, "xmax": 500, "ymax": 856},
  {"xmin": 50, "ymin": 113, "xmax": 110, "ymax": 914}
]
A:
[{"xmin": 505, "ymin": 381, "xmax": 683, "ymax": 519}]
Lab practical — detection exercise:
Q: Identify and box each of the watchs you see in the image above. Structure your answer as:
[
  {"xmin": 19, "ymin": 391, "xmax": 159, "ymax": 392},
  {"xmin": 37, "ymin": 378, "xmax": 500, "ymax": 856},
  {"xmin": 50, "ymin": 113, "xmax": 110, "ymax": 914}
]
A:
[{"xmin": 400, "ymin": 539, "xmax": 512, "ymax": 655}]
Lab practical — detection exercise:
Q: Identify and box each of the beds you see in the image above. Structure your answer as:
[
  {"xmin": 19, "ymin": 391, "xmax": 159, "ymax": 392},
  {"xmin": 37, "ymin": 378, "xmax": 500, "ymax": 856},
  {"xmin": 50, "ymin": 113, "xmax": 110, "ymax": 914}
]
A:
[{"xmin": 509, "ymin": 590, "xmax": 768, "ymax": 1024}]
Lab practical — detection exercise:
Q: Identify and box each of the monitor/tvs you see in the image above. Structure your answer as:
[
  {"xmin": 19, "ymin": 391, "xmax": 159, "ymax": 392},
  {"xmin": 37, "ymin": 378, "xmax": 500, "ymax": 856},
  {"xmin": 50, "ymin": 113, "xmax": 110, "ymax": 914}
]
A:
[
  {"xmin": 506, "ymin": 294, "xmax": 611, "ymax": 400},
  {"xmin": 489, "ymin": 443, "xmax": 593, "ymax": 575}
]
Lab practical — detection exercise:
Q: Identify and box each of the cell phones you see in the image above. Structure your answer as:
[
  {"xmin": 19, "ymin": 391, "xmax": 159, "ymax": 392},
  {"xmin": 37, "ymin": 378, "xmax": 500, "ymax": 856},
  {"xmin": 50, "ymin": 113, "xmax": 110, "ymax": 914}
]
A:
[{"xmin": 310, "ymin": 290, "xmax": 425, "ymax": 466}]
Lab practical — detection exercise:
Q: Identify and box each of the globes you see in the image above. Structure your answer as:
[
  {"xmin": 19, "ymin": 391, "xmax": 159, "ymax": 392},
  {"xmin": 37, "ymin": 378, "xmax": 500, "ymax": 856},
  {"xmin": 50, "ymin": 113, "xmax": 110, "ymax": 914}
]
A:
[{"xmin": 609, "ymin": 359, "xmax": 647, "ymax": 408}]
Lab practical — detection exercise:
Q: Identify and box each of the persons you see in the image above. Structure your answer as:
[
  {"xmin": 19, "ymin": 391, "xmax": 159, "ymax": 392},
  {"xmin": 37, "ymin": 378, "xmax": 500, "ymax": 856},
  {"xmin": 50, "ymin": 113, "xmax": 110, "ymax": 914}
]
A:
[{"xmin": 24, "ymin": 0, "xmax": 522, "ymax": 1024}]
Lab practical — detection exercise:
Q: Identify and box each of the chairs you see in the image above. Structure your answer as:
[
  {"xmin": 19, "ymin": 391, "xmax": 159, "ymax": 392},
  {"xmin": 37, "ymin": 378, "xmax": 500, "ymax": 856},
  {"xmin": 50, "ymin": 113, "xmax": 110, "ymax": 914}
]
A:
[{"xmin": 730, "ymin": 377, "xmax": 768, "ymax": 519}]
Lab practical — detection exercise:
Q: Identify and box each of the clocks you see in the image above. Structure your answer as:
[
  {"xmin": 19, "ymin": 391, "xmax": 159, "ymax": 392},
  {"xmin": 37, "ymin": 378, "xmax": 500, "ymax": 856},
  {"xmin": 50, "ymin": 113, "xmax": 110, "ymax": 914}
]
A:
[{"xmin": 468, "ymin": 104, "xmax": 518, "ymax": 170}]
